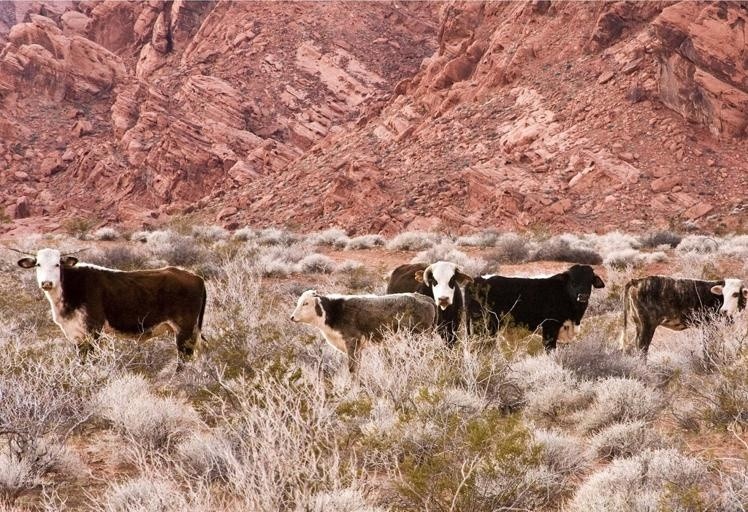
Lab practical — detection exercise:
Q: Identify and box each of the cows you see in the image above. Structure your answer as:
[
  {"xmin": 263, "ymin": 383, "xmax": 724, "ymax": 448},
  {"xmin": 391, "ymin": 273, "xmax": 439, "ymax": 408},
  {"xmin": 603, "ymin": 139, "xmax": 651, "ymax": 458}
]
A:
[
  {"xmin": 463, "ymin": 262, "xmax": 607, "ymax": 355},
  {"xmin": 620, "ymin": 273, "xmax": 748, "ymax": 364},
  {"xmin": 289, "ymin": 286, "xmax": 441, "ymax": 373},
  {"xmin": 386, "ymin": 259, "xmax": 473, "ymax": 349},
  {"xmin": 16, "ymin": 246, "xmax": 208, "ymax": 374}
]
